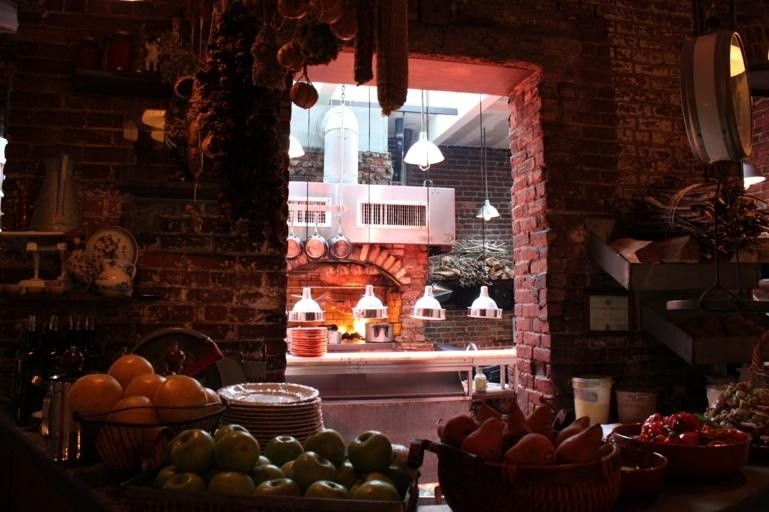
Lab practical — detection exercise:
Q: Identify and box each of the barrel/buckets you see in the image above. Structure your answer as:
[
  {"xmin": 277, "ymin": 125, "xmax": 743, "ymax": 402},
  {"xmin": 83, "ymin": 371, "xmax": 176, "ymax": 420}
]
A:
[
  {"xmin": 616, "ymin": 389, "xmax": 657, "ymax": 423},
  {"xmin": 570, "ymin": 373, "xmax": 613, "ymax": 424}
]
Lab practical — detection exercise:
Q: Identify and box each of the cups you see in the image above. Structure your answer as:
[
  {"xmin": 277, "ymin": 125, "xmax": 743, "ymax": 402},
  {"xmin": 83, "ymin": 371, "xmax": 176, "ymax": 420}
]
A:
[{"xmin": 40, "ymin": 370, "xmax": 96, "ymax": 466}]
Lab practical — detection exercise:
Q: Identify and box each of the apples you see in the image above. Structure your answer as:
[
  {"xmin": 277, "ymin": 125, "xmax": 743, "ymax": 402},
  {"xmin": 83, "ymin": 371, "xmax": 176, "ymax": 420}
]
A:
[{"xmin": 155, "ymin": 424, "xmax": 416, "ymax": 501}]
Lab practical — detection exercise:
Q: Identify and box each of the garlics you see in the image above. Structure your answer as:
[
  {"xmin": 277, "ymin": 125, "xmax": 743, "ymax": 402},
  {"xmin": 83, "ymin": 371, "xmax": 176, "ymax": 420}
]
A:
[{"xmin": 196, "ymin": 34, "xmax": 246, "ymax": 212}]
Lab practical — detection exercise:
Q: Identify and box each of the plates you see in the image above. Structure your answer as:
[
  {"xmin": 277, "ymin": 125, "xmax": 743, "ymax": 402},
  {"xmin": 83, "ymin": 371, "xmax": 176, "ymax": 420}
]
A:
[
  {"xmin": 290, "ymin": 327, "xmax": 327, "ymax": 357},
  {"xmin": 220, "ymin": 381, "xmax": 327, "ymax": 455},
  {"xmin": 83, "ymin": 223, "xmax": 140, "ymax": 272}
]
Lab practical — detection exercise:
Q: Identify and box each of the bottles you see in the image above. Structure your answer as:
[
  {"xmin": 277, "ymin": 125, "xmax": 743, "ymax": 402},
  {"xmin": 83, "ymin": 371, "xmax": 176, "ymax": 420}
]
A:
[
  {"xmin": 15, "ymin": 311, "xmax": 97, "ymax": 428},
  {"xmin": 474, "ymin": 367, "xmax": 487, "ymax": 395}
]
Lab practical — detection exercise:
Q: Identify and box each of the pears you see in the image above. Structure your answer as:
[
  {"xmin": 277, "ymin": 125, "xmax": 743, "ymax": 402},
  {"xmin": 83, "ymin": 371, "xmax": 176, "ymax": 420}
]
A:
[{"xmin": 435, "ymin": 392, "xmax": 604, "ymax": 466}]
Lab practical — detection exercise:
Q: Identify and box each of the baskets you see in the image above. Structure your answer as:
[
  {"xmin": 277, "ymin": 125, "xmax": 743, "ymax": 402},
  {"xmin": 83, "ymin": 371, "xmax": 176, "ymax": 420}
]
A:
[{"xmin": 73, "ymin": 397, "xmax": 227, "ymax": 472}]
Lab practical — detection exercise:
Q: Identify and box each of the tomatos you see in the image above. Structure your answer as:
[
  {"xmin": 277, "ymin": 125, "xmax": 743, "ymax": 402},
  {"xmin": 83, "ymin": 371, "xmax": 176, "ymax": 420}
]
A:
[{"xmin": 637, "ymin": 410, "xmax": 747, "ymax": 447}]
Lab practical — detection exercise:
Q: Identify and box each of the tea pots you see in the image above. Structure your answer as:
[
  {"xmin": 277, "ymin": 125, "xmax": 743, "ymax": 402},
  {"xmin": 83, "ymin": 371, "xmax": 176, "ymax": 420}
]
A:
[
  {"xmin": 27, "ymin": 151, "xmax": 85, "ymax": 231},
  {"xmin": 93, "ymin": 254, "xmax": 137, "ymax": 296}
]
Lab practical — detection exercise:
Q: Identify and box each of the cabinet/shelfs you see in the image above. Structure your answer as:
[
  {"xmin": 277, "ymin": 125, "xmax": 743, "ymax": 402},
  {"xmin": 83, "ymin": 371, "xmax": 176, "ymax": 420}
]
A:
[{"xmin": 0, "ymin": 229, "xmax": 163, "ymax": 307}]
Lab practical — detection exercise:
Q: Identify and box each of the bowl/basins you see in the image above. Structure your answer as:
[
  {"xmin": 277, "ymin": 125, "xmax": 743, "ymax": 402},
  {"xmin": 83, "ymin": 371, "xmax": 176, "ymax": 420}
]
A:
[
  {"xmin": 619, "ymin": 447, "xmax": 670, "ymax": 501},
  {"xmin": 750, "ymin": 441, "xmax": 769, "ymax": 461},
  {"xmin": 612, "ymin": 419, "xmax": 754, "ymax": 482}
]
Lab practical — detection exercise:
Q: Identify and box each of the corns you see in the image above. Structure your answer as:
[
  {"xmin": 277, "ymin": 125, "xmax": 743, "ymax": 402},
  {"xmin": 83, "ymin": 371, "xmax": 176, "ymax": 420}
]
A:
[{"xmin": 377, "ymin": 1, "xmax": 409, "ymax": 116}]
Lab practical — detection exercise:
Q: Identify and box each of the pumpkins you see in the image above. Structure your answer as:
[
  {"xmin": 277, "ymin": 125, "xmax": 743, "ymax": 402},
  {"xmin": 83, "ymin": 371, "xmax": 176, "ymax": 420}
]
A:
[{"xmin": 275, "ymin": 1, "xmax": 359, "ymax": 110}]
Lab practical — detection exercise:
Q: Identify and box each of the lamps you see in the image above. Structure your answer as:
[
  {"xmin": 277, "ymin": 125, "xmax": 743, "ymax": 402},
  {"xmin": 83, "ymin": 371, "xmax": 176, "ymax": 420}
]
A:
[
  {"xmin": 402, "ymin": 88, "xmax": 445, "ymax": 170},
  {"xmin": 467, "ymin": 98, "xmax": 503, "ymax": 320},
  {"xmin": 410, "ymin": 89, "xmax": 446, "ymax": 320},
  {"xmin": 289, "ymin": 136, "xmax": 304, "ymax": 158},
  {"xmin": 352, "ymin": 84, "xmax": 388, "ymax": 318},
  {"xmin": 475, "ymin": 128, "xmax": 500, "ymax": 221},
  {"xmin": 289, "ymin": 82, "xmax": 323, "ymax": 322}
]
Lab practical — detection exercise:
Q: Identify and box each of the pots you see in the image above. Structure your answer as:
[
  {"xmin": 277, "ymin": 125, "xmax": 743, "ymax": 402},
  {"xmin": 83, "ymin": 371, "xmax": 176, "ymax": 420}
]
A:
[
  {"xmin": 321, "ymin": 325, "xmax": 342, "ymax": 344},
  {"xmin": 364, "ymin": 321, "xmax": 393, "ymax": 343}
]
